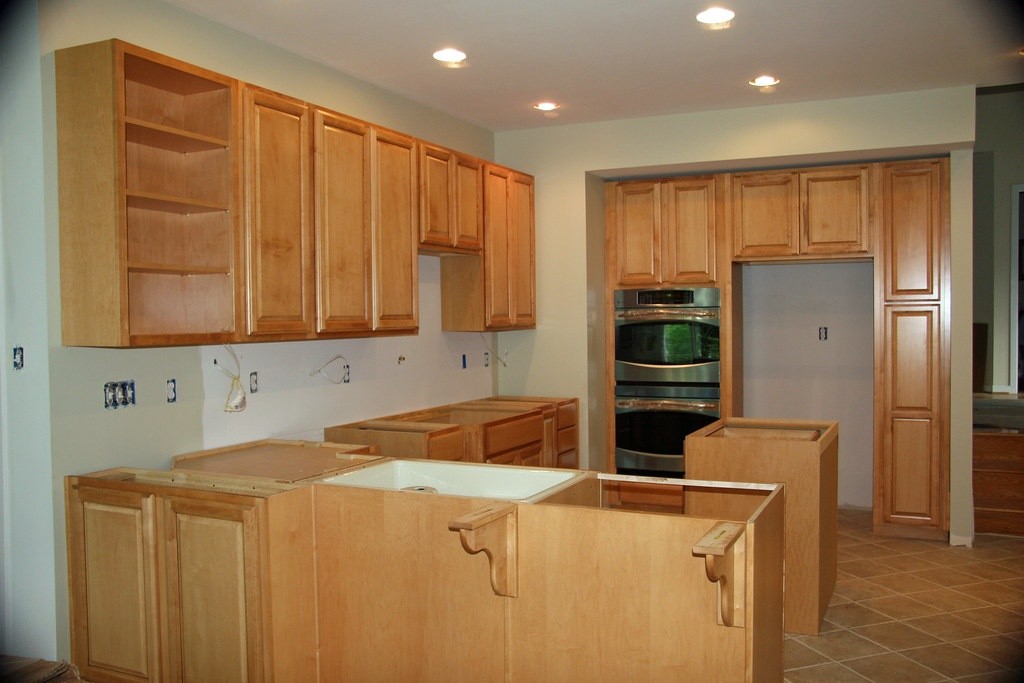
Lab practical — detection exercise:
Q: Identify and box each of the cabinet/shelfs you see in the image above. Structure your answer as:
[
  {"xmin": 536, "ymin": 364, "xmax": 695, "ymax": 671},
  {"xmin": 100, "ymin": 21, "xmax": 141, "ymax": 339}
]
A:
[
  {"xmin": 871, "ymin": 157, "xmax": 950, "ymax": 543},
  {"xmin": 732, "ymin": 164, "xmax": 873, "ymax": 263},
  {"xmin": 51, "ymin": 38, "xmax": 536, "ymax": 349},
  {"xmin": 64, "ymin": 397, "xmax": 840, "ymax": 683},
  {"xmin": 603, "ymin": 172, "xmax": 731, "ymax": 287}
]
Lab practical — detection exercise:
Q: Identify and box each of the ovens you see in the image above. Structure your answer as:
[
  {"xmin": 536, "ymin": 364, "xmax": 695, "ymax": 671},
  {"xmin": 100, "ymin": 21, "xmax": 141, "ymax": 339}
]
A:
[
  {"xmin": 615, "ymin": 406, "xmax": 720, "ymax": 478},
  {"xmin": 614, "ymin": 288, "xmax": 720, "ymax": 399}
]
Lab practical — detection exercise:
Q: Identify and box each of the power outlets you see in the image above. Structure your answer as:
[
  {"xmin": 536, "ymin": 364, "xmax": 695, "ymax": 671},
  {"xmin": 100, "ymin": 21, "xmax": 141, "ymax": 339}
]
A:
[
  {"xmin": 818, "ymin": 326, "xmax": 828, "ymax": 340},
  {"xmin": 250, "ymin": 372, "xmax": 259, "ymax": 394},
  {"xmin": 485, "ymin": 353, "xmax": 489, "ymax": 367},
  {"xmin": 13, "ymin": 346, "xmax": 25, "ymax": 369},
  {"xmin": 104, "ymin": 380, "xmax": 135, "ymax": 410},
  {"xmin": 343, "ymin": 365, "xmax": 350, "ymax": 384},
  {"xmin": 166, "ymin": 379, "xmax": 176, "ymax": 402}
]
